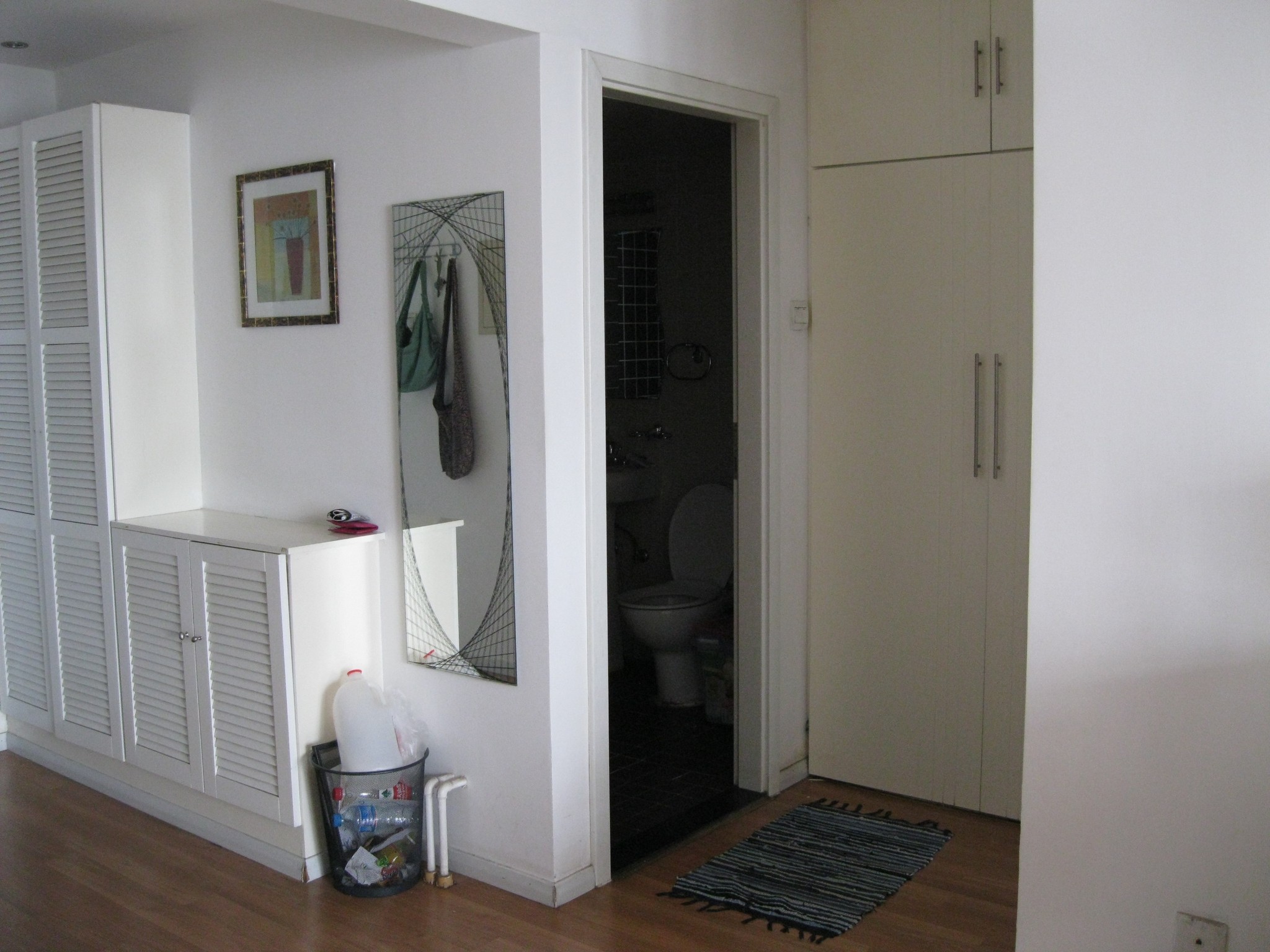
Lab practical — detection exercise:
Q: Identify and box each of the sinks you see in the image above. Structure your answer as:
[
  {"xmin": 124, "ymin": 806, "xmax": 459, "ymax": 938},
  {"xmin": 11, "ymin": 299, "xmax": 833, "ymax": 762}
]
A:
[{"xmin": 607, "ymin": 466, "xmax": 656, "ymax": 502}]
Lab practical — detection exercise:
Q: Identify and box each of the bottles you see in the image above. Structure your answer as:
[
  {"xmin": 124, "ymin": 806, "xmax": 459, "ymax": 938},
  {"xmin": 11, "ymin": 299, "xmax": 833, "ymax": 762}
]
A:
[
  {"xmin": 374, "ymin": 830, "xmax": 420, "ymax": 888},
  {"xmin": 333, "ymin": 783, "xmax": 424, "ymax": 806},
  {"xmin": 330, "ymin": 851, "xmax": 356, "ymax": 876},
  {"xmin": 332, "ymin": 799, "xmax": 423, "ymax": 836},
  {"xmin": 332, "ymin": 669, "xmax": 405, "ymax": 798}
]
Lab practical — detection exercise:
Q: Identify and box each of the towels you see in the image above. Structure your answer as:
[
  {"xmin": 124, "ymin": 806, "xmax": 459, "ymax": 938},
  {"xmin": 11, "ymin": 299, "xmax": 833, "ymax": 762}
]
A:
[{"xmin": 604, "ymin": 229, "xmax": 666, "ymax": 401}]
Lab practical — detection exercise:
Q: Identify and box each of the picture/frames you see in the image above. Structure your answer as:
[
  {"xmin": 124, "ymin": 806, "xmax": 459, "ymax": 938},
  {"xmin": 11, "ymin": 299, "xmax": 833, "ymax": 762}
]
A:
[{"xmin": 234, "ymin": 158, "xmax": 346, "ymax": 330}]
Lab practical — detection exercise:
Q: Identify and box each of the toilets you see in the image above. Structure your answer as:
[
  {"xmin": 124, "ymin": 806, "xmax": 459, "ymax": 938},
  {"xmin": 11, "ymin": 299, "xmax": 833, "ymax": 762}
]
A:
[{"xmin": 616, "ymin": 484, "xmax": 734, "ymax": 707}]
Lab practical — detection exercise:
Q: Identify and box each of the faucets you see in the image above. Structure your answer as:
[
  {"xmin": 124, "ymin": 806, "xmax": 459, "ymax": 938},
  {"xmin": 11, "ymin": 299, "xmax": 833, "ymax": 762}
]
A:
[{"xmin": 606, "ymin": 442, "xmax": 627, "ymax": 466}]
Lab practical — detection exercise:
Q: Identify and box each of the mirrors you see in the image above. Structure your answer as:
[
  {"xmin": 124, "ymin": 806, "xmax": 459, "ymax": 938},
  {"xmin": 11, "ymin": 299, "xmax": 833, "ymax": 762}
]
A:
[{"xmin": 389, "ymin": 188, "xmax": 522, "ymax": 690}]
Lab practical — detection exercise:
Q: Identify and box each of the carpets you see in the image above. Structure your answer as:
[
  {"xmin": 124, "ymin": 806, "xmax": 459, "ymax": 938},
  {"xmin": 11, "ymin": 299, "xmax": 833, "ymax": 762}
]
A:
[{"xmin": 665, "ymin": 799, "xmax": 957, "ymax": 946}]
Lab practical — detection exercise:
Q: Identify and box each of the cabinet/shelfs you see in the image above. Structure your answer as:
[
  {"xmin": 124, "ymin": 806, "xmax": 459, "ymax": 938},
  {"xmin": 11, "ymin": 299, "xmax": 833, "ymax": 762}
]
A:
[
  {"xmin": 802, "ymin": 0, "xmax": 1037, "ymax": 832},
  {"xmin": 0, "ymin": 105, "xmax": 366, "ymax": 881}
]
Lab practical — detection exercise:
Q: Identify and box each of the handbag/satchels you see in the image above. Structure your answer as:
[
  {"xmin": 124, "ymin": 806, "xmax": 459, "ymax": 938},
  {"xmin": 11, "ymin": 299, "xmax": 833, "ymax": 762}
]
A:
[
  {"xmin": 432, "ymin": 259, "xmax": 475, "ymax": 479},
  {"xmin": 395, "ymin": 258, "xmax": 440, "ymax": 393}
]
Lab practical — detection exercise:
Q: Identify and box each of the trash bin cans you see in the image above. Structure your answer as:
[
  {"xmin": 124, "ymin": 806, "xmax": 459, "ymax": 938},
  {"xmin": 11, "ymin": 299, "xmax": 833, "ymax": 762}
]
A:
[{"xmin": 310, "ymin": 738, "xmax": 430, "ymax": 897}]
